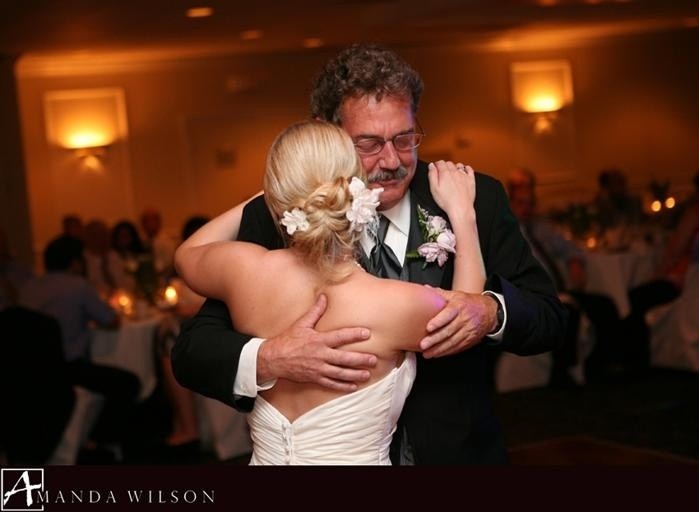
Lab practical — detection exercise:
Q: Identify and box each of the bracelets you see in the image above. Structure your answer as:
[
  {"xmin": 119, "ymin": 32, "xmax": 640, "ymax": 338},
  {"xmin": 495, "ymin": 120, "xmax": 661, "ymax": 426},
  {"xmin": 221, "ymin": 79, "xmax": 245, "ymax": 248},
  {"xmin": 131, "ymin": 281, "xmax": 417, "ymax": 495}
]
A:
[{"xmin": 489, "ymin": 296, "xmax": 503, "ymax": 336}]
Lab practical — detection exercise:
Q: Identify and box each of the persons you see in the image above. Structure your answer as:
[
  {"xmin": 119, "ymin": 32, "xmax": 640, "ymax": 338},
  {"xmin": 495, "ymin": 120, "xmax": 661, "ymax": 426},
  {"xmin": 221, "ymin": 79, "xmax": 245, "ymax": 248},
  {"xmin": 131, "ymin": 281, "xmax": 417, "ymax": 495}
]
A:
[
  {"xmin": 171, "ymin": 41, "xmax": 559, "ymax": 467},
  {"xmin": 174, "ymin": 116, "xmax": 488, "ymax": 467}
]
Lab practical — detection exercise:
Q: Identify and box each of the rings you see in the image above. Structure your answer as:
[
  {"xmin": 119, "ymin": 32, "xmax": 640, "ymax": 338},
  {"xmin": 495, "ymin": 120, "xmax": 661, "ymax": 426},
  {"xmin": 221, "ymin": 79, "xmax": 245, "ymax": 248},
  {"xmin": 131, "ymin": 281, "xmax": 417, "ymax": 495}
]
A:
[{"xmin": 458, "ymin": 166, "xmax": 466, "ymax": 170}]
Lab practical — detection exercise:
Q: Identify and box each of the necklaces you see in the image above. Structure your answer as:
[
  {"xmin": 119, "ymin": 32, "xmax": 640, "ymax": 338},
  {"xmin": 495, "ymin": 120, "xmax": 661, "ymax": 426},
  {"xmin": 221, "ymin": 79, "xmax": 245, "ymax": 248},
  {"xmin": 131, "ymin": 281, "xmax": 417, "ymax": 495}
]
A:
[{"xmin": 324, "ymin": 254, "xmax": 366, "ymax": 272}]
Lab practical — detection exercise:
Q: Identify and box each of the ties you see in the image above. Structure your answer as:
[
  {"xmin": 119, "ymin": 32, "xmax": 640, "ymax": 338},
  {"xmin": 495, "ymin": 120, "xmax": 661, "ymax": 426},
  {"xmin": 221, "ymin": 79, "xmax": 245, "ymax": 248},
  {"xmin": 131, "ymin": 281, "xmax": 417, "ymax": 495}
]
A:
[{"xmin": 368, "ymin": 216, "xmax": 402, "ymax": 278}]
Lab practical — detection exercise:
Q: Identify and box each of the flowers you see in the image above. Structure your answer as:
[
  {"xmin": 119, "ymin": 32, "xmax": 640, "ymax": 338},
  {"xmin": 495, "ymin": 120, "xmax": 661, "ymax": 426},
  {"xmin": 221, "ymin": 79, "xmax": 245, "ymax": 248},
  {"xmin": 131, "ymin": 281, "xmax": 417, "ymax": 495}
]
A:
[{"xmin": 413, "ymin": 205, "xmax": 460, "ymax": 266}]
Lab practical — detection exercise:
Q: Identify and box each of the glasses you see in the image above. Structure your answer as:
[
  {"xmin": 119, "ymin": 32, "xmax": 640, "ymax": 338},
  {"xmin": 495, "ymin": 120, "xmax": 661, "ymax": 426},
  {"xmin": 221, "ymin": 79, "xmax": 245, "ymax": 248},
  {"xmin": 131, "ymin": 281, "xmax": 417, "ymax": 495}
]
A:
[{"xmin": 352, "ymin": 115, "xmax": 425, "ymax": 156}]
